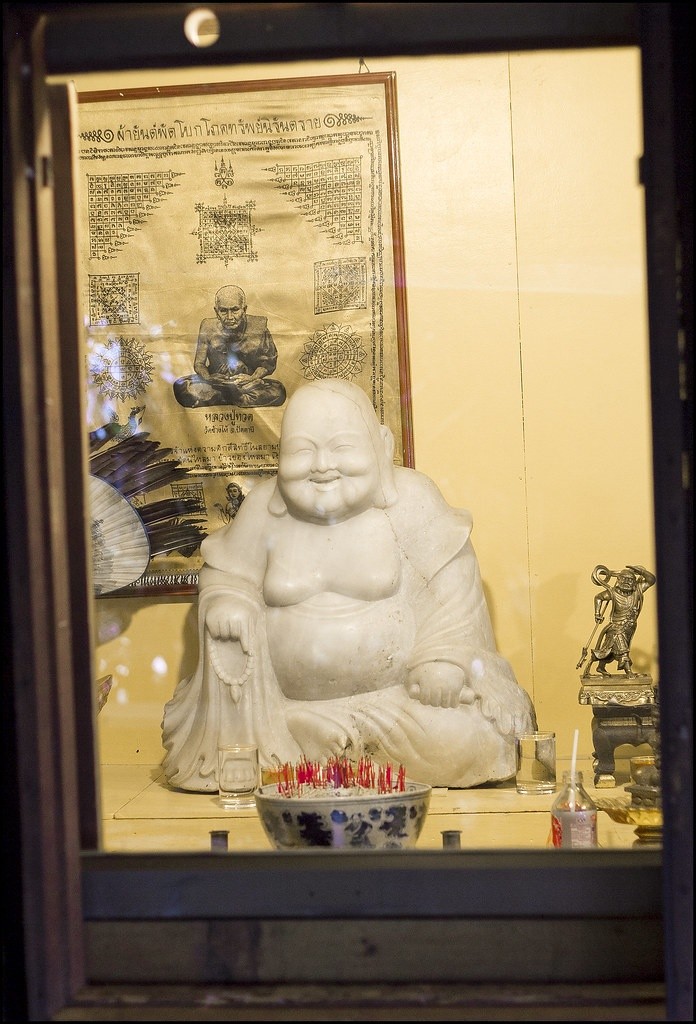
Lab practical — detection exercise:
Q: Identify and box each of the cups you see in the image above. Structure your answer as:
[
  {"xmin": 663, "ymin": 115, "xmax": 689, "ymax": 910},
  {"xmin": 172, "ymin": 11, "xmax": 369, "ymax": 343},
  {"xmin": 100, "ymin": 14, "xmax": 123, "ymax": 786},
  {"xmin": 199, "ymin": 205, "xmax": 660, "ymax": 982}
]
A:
[
  {"xmin": 516, "ymin": 732, "xmax": 558, "ymax": 795},
  {"xmin": 220, "ymin": 744, "xmax": 260, "ymax": 812}
]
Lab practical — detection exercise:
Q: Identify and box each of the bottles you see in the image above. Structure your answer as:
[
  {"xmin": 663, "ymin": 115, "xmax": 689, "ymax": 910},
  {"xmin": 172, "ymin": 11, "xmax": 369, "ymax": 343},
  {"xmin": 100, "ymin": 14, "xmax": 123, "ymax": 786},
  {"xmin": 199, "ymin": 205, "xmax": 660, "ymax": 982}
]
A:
[{"xmin": 547, "ymin": 770, "xmax": 598, "ymax": 850}]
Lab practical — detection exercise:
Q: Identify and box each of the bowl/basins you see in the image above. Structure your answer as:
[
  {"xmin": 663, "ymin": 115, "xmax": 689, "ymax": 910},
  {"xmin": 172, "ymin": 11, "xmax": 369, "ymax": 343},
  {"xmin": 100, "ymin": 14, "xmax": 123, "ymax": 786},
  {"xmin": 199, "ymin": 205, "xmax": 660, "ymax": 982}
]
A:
[{"xmin": 255, "ymin": 780, "xmax": 432, "ymax": 851}]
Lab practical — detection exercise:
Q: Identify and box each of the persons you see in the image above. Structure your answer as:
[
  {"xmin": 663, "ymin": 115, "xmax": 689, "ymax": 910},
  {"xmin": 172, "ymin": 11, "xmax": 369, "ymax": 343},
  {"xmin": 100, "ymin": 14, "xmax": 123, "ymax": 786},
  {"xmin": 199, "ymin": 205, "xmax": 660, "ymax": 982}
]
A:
[
  {"xmin": 159, "ymin": 379, "xmax": 537, "ymax": 792},
  {"xmin": 575, "ymin": 565, "xmax": 657, "ymax": 677}
]
